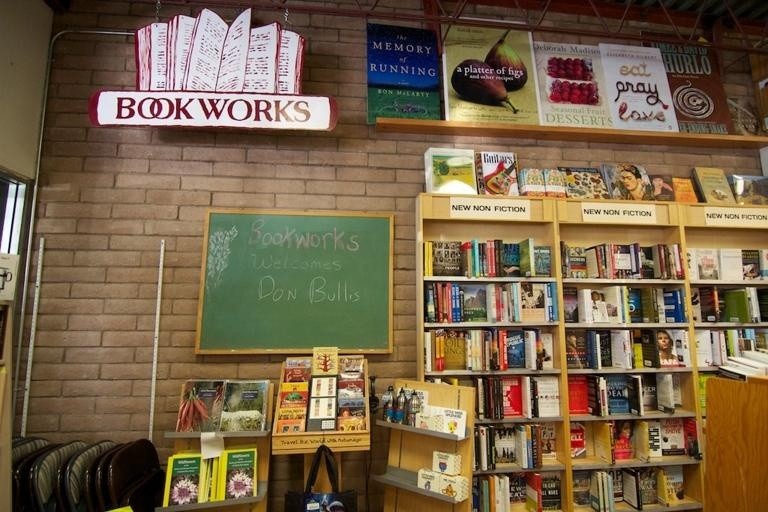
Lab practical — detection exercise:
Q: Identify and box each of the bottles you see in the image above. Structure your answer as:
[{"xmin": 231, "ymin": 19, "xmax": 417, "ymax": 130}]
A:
[
  {"xmin": 446, "ymin": 483, "xmax": 453, "ymax": 496},
  {"xmin": 438, "ymin": 450, "xmax": 447, "ymax": 472},
  {"xmin": 284, "ymin": 387, "xmax": 303, "ymax": 400},
  {"xmin": 382, "ymin": 385, "xmax": 420, "ymax": 427}
]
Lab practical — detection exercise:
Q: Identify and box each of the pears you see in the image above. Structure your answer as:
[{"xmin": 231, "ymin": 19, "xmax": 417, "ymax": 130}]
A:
[
  {"xmin": 484, "ymin": 29, "xmax": 528, "ymax": 93},
  {"xmin": 450, "ymin": 59, "xmax": 519, "ymax": 114}
]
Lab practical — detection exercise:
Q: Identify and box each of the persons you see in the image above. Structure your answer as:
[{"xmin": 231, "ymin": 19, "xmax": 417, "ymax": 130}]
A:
[{"xmin": 276, "ymin": 345, "xmax": 366, "ymax": 431}]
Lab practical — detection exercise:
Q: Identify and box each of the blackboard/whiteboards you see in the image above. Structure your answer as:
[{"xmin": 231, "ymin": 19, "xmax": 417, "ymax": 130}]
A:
[{"xmin": 195, "ymin": 208, "xmax": 395, "ymax": 355}]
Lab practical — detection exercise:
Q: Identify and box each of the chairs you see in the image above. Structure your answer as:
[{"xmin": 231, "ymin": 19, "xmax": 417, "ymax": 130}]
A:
[{"xmin": 8, "ymin": 427, "xmax": 164, "ymax": 512}]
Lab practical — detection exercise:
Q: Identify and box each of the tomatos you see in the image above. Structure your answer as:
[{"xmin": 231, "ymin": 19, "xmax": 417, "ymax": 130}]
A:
[{"xmin": 547, "ymin": 57, "xmax": 598, "ymax": 105}]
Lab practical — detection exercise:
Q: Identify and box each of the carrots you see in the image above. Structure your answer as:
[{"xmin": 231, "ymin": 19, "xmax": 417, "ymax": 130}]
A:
[{"xmin": 178, "ymin": 397, "xmax": 210, "ymax": 431}]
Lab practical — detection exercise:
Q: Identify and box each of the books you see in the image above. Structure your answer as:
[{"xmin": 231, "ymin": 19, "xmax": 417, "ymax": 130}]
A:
[{"xmin": 134, "ymin": 7, "xmax": 303, "ymax": 95}]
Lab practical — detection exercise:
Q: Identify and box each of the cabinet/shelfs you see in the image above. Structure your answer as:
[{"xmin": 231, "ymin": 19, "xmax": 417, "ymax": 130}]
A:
[
  {"xmin": 412, "ymin": 190, "xmax": 572, "ymax": 511},
  {"xmin": 677, "ymin": 200, "xmax": 768, "ymax": 477},
  {"xmin": 550, "ymin": 196, "xmax": 712, "ymax": 512},
  {"xmin": 153, "ymin": 376, "xmax": 277, "ymax": 511},
  {"xmin": 369, "ymin": 373, "xmax": 478, "ymax": 511},
  {"xmin": 701, "ymin": 373, "xmax": 768, "ymax": 512}
]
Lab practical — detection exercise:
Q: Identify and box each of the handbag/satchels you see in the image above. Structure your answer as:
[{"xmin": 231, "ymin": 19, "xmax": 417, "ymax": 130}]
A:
[{"xmin": 284, "ymin": 490, "xmax": 357, "ymax": 512}]
